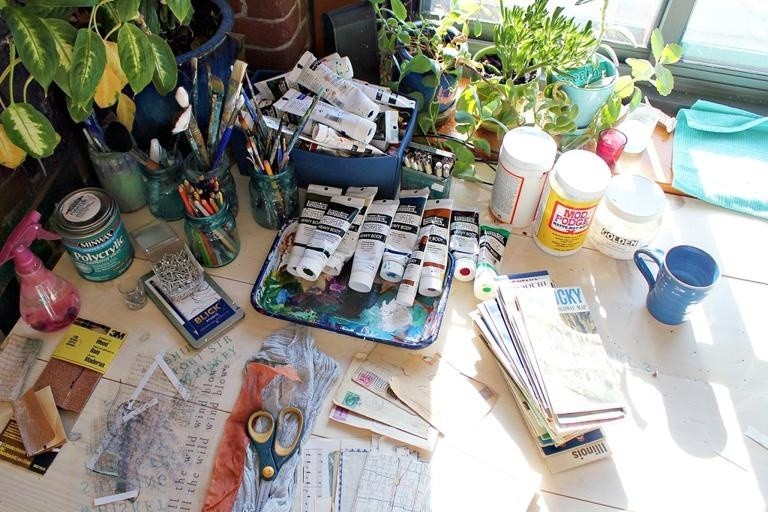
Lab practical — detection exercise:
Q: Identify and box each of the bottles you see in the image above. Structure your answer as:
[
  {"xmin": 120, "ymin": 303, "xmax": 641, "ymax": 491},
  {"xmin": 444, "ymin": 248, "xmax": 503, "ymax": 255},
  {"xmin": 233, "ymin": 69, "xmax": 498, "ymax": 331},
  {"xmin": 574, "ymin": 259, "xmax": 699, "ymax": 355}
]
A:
[
  {"xmin": 86, "ymin": 144, "xmax": 298, "ymax": 268},
  {"xmin": 490, "ymin": 126, "xmax": 666, "ymax": 258}
]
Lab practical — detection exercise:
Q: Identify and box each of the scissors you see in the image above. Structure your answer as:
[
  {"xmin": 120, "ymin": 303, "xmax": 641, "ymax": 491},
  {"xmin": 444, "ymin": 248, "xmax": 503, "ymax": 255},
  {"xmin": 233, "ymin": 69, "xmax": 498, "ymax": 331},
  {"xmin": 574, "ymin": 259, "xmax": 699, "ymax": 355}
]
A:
[{"xmin": 247, "ymin": 407, "xmax": 305, "ymax": 512}]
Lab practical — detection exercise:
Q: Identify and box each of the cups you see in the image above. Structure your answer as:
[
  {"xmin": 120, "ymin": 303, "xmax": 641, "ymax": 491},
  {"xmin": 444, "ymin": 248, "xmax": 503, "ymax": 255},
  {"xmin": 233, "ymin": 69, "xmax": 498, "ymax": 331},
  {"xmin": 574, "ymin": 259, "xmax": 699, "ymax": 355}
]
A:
[
  {"xmin": 633, "ymin": 244, "xmax": 721, "ymax": 326},
  {"xmin": 618, "ymin": 103, "xmax": 658, "ymax": 154}
]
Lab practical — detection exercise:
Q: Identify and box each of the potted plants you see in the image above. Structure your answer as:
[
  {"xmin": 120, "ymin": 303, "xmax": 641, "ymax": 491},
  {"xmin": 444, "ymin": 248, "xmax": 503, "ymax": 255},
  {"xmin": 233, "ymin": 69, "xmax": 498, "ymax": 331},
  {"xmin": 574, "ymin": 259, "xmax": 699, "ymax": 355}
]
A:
[
  {"xmin": 0, "ymin": 1, "xmax": 233, "ymax": 159},
  {"xmin": 470, "ymin": 1, "xmax": 594, "ymax": 131},
  {"xmin": 373, "ymin": 0, "xmax": 469, "ymax": 124},
  {"xmin": 552, "ymin": 0, "xmax": 679, "ymax": 136}
]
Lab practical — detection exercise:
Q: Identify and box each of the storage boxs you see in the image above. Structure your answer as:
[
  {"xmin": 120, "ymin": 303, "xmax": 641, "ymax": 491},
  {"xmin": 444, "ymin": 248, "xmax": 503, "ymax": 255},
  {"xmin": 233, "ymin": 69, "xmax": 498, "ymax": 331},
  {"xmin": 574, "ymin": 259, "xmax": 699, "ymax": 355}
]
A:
[{"xmin": 294, "ymin": 93, "xmax": 420, "ymax": 200}]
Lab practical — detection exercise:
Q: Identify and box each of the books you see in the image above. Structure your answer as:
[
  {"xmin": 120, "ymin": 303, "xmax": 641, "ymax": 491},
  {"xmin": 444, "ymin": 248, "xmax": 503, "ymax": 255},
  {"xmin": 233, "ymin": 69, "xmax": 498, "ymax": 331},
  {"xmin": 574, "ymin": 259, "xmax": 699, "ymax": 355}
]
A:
[{"xmin": 10, "ymin": 385, "xmax": 68, "ymax": 457}]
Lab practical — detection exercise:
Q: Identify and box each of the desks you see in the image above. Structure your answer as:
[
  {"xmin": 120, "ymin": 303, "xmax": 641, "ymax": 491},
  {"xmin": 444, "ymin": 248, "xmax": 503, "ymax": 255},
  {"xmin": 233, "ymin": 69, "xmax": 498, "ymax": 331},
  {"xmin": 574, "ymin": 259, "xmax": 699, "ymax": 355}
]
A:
[{"xmin": 1, "ymin": 150, "xmax": 764, "ymax": 511}]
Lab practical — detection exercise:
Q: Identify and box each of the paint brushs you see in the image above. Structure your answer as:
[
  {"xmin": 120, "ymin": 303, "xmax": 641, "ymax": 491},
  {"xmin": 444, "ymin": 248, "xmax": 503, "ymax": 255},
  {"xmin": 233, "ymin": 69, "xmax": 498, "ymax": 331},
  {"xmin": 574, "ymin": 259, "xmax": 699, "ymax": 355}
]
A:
[
  {"xmin": 175, "ymin": 56, "xmax": 246, "ymax": 170},
  {"xmin": 104, "ymin": 121, "xmax": 165, "ymax": 170}
]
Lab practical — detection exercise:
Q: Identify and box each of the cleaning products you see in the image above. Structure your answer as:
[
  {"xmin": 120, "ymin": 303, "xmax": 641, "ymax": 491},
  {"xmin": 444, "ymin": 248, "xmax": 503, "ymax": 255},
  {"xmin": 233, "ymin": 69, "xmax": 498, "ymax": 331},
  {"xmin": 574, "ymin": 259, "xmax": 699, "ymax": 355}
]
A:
[{"xmin": 1, "ymin": 209, "xmax": 80, "ymax": 333}]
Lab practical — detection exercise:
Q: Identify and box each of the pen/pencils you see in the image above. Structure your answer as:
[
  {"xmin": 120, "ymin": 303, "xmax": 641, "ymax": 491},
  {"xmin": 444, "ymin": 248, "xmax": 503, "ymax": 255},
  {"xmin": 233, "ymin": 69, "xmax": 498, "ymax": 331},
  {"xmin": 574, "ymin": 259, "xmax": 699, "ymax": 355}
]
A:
[
  {"xmin": 239, "ymin": 72, "xmax": 327, "ymax": 228},
  {"xmin": 148, "ymin": 138, "xmax": 163, "ymax": 214},
  {"xmin": 178, "ymin": 173, "xmax": 239, "ymax": 268}
]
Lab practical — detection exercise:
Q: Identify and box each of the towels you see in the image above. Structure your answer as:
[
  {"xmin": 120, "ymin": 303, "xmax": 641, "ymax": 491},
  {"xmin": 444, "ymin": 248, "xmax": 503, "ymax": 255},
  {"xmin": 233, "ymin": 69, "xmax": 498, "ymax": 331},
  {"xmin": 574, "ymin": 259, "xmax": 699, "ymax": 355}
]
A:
[{"xmin": 670, "ymin": 99, "xmax": 768, "ymax": 219}]
